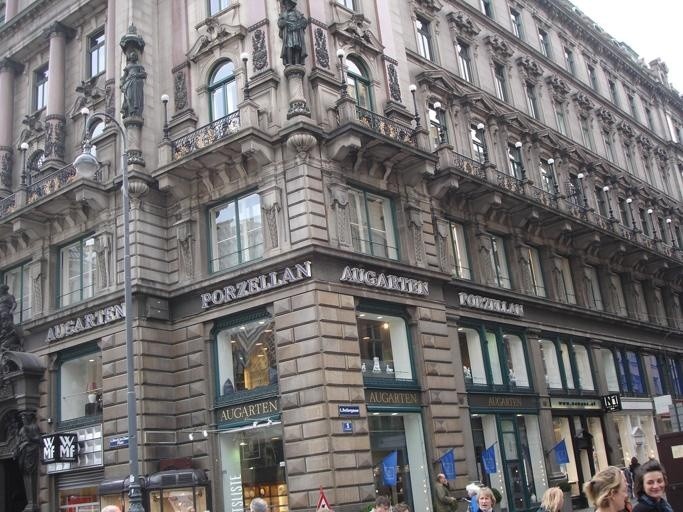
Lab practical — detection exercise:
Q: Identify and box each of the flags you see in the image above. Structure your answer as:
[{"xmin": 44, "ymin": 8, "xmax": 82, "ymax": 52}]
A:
[
  {"xmin": 379, "ymin": 450, "xmax": 398, "ymax": 487},
  {"xmin": 480, "ymin": 445, "xmax": 496, "ymax": 474},
  {"xmin": 436, "ymin": 448, "xmax": 455, "ymax": 480},
  {"xmin": 552, "ymin": 438, "xmax": 570, "ymax": 466}
]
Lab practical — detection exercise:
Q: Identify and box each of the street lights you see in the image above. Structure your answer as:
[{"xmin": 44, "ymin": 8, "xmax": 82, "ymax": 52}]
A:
[{"xmin": 72, "ymin": 112, "xmax": 145, "ymax": 511}]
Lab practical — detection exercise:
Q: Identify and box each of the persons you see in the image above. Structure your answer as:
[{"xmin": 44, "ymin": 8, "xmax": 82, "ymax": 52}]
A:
[
  {"xmin": 276, "ymin": 0, "xmax": 309, "ymax": 66},
  {"xmin": 101, "ymin": 504, "xmax": 123, "ymax": 512},
  {"xmin": 9, "ymin": 410, "xmax": 42, "ymax": 512},
  {"xmin": 534, "ymin": 485, "xmax": 566, "ymax": 512},
  {"xmin": 119, "ymin": 48, "xmax": 147, "ymax": 121},
  {"xmin": 461, "ymin": 482, "xmax": 480, "ymax": 512},
  {"xmin": 0, "ymin": 283, "xmax": 18, "ymax": 333},
  {"xmin": 477, "ymin": 487, "xmax": 497, "ymax": 512},
  {"xmin": 367, "ymin": 497, "xmax": 390, "ymax": 512},
  {"xmin": 430, "ymin": 474, "xmax": 456, "ymax": 512},
  {"xmin": 391, "ymin": 502, "xmax": 411, "ymax": 512},
  {"xmin": 585, "ymin": 449, "xmax": 675, "ymax": 512},
  {"xmin": 248, "ymin": 498, "xmax": 267, "ymax": 512}
]
